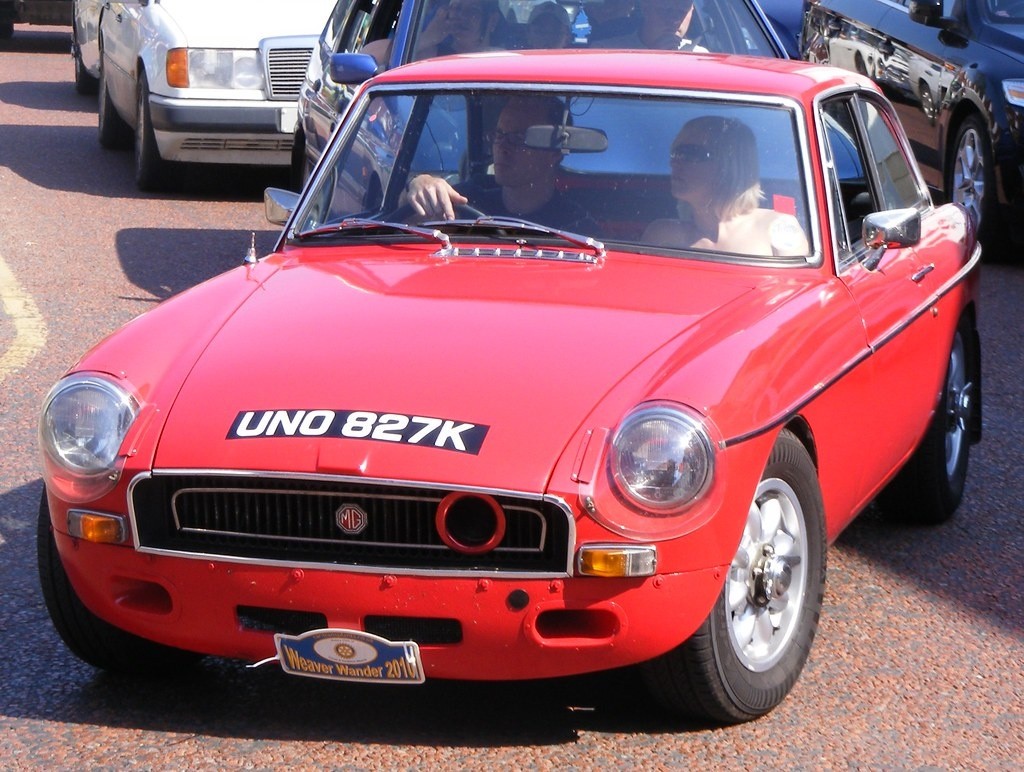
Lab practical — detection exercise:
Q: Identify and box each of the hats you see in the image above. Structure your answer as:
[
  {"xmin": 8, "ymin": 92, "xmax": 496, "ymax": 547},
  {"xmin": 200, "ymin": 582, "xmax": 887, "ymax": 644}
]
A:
[{"xmin": 527, "ymin": 1, "xmax": 571, "ymax": 29}]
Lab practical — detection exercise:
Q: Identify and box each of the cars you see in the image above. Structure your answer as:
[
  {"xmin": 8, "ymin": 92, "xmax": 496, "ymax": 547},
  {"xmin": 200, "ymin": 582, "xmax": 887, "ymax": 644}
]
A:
[
  {"xmin": 36, "ymin": 51, "xmax": 984, "ymax": 725},
  {"xmin": 741, "ymin": 1, "xmax": 1023, "ymax": 263},
  {"xmin": 0, "ymin": 0, "xmax": 345, "ymax": 196},
  {"xmin": 288, "ymin": 1, "xmax": 876, "ymax": 254}
]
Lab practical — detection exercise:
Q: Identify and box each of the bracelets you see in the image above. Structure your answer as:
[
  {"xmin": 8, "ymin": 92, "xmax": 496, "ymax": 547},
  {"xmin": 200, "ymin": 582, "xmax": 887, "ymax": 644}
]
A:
[{"xmin": 405, "ymin": 174, "xmax": 417, "ymax": 192}]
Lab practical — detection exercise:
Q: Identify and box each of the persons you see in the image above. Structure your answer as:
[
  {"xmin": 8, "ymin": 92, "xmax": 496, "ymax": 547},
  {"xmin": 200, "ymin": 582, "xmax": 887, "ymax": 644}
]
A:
[
  {"xmin": 641, "ymin": 118, "xmax": 811, "ymax": 257},
  {"xmin": 361, "ymin": 0, "xmax": 511, "ymax": 64},
  {"xmin": 589, "ymin": 0, "xmax": 711, "ymax": 54},
  {"xmin": 526, "ymin": 1, "xmax": 571, "ymax": 49},
  {"xmin": 397, "ymin": 94, "xmax": 604, "ymax": 238}
]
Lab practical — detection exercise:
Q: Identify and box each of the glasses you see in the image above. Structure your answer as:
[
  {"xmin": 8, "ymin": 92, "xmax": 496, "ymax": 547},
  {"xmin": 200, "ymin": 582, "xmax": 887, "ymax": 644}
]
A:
[
  {"xmin": 485, "ymin": 131, "xmax": 525, "ymax": 146},
  {"xmin": 670, "ymin": 144, "xmax": 722, "ymax": 163}
]
